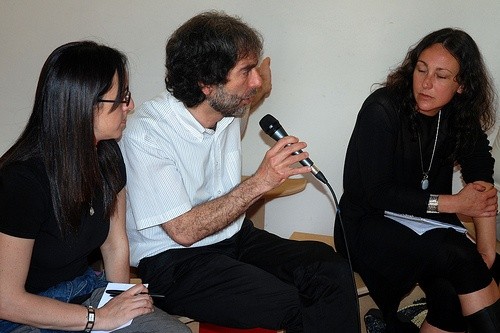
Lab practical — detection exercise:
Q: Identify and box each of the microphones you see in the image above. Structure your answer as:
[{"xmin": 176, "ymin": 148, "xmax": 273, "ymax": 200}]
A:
[{"xmin": 259, "ymin": 114, "xmax": 328, "ymax": 184}]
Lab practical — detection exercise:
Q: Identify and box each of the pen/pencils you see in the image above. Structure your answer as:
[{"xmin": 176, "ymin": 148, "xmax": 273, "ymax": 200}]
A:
[{"xmin": 106, "ymin": 288, "xmax": 165, "ymax": 300}]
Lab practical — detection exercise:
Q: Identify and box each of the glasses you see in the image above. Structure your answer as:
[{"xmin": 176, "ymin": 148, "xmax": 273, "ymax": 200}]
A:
[{"xmin": 100, "ymin": 91, "xmax": 131, "ymax": 107}]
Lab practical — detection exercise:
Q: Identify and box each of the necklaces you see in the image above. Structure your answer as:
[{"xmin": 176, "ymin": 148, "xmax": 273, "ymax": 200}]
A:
[{"xmin": 416, "ymin": 111, "xmax": 442, "ymax": 191}]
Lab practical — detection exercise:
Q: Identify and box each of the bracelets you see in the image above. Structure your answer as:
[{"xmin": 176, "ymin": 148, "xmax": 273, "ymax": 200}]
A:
[
  {"xmin": 427, "ymin": 194, "xmax": 438, "ymax": 214},
  {"xmin": 84, "ymin": 305, "xmax": 96, "ymax": 331}
]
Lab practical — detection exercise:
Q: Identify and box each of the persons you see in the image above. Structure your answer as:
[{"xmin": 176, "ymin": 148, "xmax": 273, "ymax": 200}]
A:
[
  {"xmin": 0, "ymin": 42, "xmax": 193, "ymax": 333},
  {"xmin": 334, "ymin": 29, "xmax": 500, "ymax": 333},
  {"xmin": 119, "ymin": 12, "xmax": 360, "ymax": 333}
]
